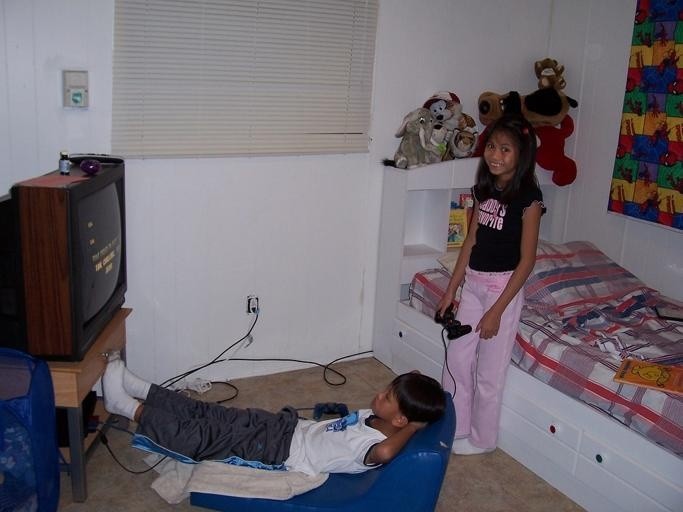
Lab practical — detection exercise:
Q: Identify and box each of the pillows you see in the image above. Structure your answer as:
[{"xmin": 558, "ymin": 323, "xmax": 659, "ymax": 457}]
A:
[{"xmin": 437, "ymin": 248, "xmax": 461, "ymax": 275}]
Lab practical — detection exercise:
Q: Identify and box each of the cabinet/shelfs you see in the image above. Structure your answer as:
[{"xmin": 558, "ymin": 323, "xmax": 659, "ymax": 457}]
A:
[{"xmin": 45, "ymin": 308, "xmax": 132, "ymax": 504}]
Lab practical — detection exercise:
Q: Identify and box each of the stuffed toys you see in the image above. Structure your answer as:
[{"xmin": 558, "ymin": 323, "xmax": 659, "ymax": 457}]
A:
[
  {"xmin": 478, "ymin": 88, "xmax": 578, "ymax": 127},
  {"xmin": 534, "ymin": 58, "xmax": 565, "ymax": 89},
  {"xmin": 394, "ymin": 91, "xmax": 478, "ymax": 169},
  {"xmin": 474, "ymin": 115, "xmax": 577, "ymax": 186}
]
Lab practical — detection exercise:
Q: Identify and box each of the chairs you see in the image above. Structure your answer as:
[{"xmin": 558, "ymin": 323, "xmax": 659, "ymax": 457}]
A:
[
  {"xmin": 0, "ymin": 347, "xmax": 60, "ymax": 512},
  {"xmin": 189, "ymin": 392, "xmax": 456, "ymax": 511}
]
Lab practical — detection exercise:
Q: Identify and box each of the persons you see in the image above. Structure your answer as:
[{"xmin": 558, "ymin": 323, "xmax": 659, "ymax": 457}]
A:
[
  {"xmin": 101, "ymin": 353, "xmax": 447, "ymax": 475},
  {"xmin": 441, "ymin": 111, "xmax": 547, "ymax": 457}
]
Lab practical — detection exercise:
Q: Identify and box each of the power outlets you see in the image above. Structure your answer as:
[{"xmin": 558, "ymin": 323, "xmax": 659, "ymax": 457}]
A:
[{"xmin": 247, "ymin": 295, "xmax": 258, "ymax": 314}]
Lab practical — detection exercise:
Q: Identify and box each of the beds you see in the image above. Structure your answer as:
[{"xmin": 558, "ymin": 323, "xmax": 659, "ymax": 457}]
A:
[{"xmin": 370, "ymin": 157, "xmax": 682, "ymax": 511}]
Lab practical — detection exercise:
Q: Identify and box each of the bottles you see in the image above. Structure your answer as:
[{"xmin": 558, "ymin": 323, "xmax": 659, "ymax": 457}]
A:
[{"xmin": 59, "ymin": 151, "xmax": 72, "ymax": 175}]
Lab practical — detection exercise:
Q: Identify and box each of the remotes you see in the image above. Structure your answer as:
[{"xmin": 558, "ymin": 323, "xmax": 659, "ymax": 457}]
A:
[{"xmin": 69, "ymin": 153, "xmax": 124, "ymax": 164}]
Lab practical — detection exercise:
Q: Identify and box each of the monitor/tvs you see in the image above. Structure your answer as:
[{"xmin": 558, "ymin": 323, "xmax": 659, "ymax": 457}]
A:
[{"xmin": 0, "ymin": 165, "xmax": 127, "ymax": 362}]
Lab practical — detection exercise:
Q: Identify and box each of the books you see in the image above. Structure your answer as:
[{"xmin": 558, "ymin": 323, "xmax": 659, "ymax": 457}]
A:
[{"xmin": 613, "ymin": 358, "xmax": 682, "ymax": 395}]
